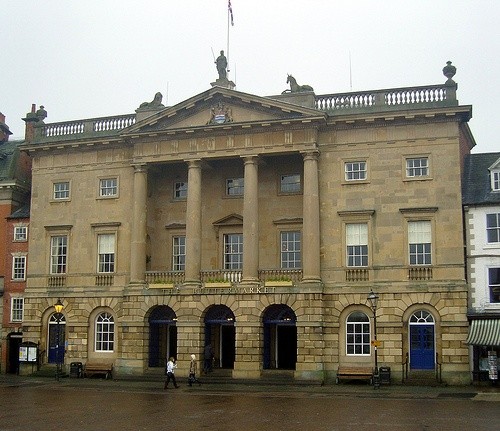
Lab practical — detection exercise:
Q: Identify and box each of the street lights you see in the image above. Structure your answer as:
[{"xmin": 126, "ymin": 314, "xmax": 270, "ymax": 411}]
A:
[
  {"xmin": 366, "ymin": 288, "xmax": 381, "ymax": 391},
  {"xmin": 53, "ymin": 298, "xmax": 64, "ymax": 381}
]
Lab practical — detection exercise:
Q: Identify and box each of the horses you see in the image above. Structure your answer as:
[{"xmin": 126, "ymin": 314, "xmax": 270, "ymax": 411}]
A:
[{"xmin": 282, "ymin": 74, "xmax": 313, "ymax": 94}]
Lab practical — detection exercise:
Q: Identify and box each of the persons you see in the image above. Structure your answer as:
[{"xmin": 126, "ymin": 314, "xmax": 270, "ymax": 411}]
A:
[
  {"xmin": 164, "ymin": 357, "xmax": 180, "ymax": 389},
  {"xmin": 204, "ymin": 342, "xmax": 214, "ymax": 374},
  {"xmin": 187, "ymin": 355, "xmax": 201, "ymax": 387},
  {"xmin": 215, "ymin": 50, "xmax": 228, "ymax": 79}
]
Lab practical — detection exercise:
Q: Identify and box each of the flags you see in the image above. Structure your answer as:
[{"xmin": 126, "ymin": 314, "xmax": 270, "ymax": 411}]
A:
[{"xmin": 228, "ymin": 0, "xmax": 234, "ymax": 26}]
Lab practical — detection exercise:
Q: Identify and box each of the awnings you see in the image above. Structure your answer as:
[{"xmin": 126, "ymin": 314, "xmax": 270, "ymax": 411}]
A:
[{"xmin": 463, "ymin": 319, "xmax": 500, "ymax": 346}]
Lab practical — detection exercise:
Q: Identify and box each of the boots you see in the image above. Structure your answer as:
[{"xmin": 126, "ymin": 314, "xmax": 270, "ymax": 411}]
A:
[
  {"xmin": 188, "ymin": 382, "xmax": 192, "ymax": 386},
  {"xmin": 197, "ymin": 379, "xmax": 201, "ymax": 386}
]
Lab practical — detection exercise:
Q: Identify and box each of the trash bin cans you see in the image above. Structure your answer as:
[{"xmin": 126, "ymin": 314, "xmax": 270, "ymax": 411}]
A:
[
  {"xmin": 380, "ymin": 367, "xmax": 391, "ymax": 384},
  {"xmin": 70, "ymin": 362, "xmax": 83, "ymax": 377}
]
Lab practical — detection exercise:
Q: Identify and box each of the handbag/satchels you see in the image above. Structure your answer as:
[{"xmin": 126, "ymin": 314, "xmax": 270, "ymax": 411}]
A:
[{"xmin": 164, "ymin": 368, "xmax": 167, "ymax": 375}]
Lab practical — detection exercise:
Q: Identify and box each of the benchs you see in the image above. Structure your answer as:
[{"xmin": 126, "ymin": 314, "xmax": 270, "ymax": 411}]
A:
[
  {"xmin": 81, "ymin": 362, "xmax": 113, "ymax": 380},
  {"xmin": 336, "ymin": 366, "xmax": 374, "ymax": 385}
]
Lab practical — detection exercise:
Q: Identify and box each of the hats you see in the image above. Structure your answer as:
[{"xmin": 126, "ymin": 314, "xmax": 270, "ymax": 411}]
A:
[{"xmin": 191, "ymin": 354, "xmax": 196, "ymax": 360}]
